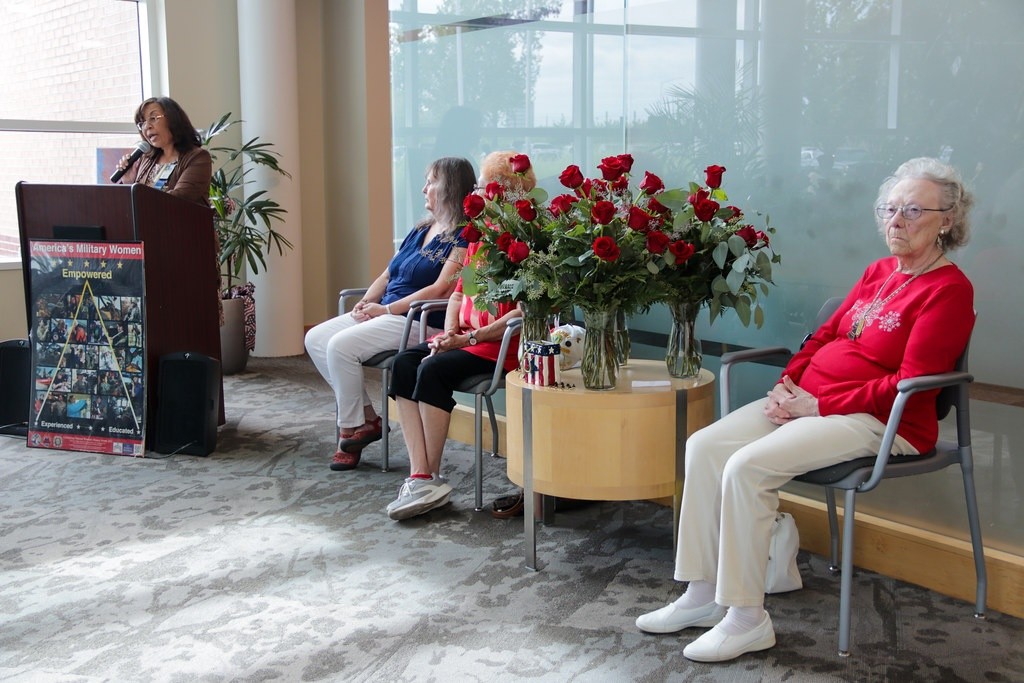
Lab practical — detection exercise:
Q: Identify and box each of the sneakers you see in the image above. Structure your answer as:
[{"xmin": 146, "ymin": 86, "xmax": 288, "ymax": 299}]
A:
[
  {"xmin": 684, "ymin": 609, "xmax": 776, "ymax": 662},
  {"xmin": 635, "ymin": 599, "xmax": 728, "ymax": 634},
  {"xmin": 385, "ymin": 473, "xmax": 453, "ymax": 521}
]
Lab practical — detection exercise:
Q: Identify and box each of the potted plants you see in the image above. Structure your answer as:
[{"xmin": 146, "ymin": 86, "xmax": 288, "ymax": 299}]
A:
[{"xmin": 197, "ymin": 111, "xmax": 295, "ymax": 375}]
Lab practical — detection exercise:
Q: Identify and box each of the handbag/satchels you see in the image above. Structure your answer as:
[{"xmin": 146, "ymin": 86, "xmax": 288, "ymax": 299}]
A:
[
  {"xmin": 764, "ymin": 511, "xmax": 804, "ymax": 595},
  {"xmin": 491, "ymin": 489, "xmax": 600, "ymax": 518}
]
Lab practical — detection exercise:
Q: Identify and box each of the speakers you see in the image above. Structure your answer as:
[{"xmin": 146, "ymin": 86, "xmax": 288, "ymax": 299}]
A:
[
  {"xmin": 153, "ymin": 351, "xmax": 220, "ymax": 458},
  {"xmin": 0, "ymin": 339, "xmax": 30, "ymax": 437}
]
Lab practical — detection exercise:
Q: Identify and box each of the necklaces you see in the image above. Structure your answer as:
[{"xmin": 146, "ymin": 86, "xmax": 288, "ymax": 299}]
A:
[{"xmin": 847, "ymin": 248, "xmax": 944, "ymax": 341}]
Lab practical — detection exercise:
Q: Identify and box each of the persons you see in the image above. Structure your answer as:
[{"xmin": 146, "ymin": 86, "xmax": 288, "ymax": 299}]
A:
[
  {"xmin": 635, "ymin": 158, "xmax": 976, "ymax": 663},
  {"xmin": 305, "ymin": 158, "xmax": 477, "ymax": 470},
  {"xmin": 116, "ymin": 97, "xmax": 226, "ymax": 432},
  {"xmin": 386, "ymin": 151, "xmax": 537, "ymax": 520},
  {"xmin": 35, "ymin": 293, "xmax": 143, "ymax": 423}
]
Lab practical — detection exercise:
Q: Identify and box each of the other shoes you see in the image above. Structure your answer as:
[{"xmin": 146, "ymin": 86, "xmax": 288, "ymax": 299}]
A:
[{"xmin": 330, "ymin": 416, "xmax": 391, "ymax": 470}]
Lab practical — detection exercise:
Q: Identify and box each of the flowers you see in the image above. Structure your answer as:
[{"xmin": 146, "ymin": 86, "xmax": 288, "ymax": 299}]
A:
[{"xmin": 418, "ymin": 152, "xmax": 781, "ymax": 387}]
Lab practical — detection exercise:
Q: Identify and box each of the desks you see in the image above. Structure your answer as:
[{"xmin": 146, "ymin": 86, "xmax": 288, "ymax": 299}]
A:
[{"xmin": 507, "ymin": 359, "xmax": 717, "ymax": 572}]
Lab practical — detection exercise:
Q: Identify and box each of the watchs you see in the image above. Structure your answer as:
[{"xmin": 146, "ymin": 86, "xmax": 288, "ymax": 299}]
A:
[{"xmin": 469, "ymin": 332, "xmax": 478, "ymax": 347}]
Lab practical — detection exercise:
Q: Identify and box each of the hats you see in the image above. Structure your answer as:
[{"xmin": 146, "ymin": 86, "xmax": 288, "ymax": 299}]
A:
[{"xmin": 77, "ymin": 369, "xmax": 84, "ymax": 375}]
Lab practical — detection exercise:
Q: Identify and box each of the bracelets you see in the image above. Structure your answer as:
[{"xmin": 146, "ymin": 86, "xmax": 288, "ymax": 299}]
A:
[
  {"xmin": 361, "ymin": 298, "xmax": 369, "ymax": 304},
  {"xmin": 386, "ymin": 304, "xmax": 390, "ymax": 314}
]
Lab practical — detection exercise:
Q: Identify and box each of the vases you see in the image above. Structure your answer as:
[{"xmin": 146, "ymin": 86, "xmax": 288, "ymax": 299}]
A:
[
  {"xmin": 616, "ymin": 307, "xmax": 631, "ymax": 366},
  {"xmin": 583, "ymin": 309, "xmax": 619, "ymax": 390},
  {"xmin": 517, "ymin": 302, "xmax": 553, "ymax": 371},
  {"xmin": 665, "ymin": 302, "xmax": 703, "ymax": 379}
]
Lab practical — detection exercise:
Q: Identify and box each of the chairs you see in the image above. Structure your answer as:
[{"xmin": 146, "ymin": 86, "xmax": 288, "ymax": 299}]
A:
[
  {"xmin": 339, "ymin": 288, "xmax": 585, "ymax": 511},
  {"xmin": 720, "ymin": 294, "xmax": 986, "ymax": 658}
]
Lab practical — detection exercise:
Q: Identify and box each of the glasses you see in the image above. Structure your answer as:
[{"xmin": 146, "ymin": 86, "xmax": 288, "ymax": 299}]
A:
[
  {"xmin": 472, "ymin": 183, "xmax": 486, "ymax": 193},
  {"xmin": 875, "ymin": 201, "xmax": 950, "ymax": 218},
  {"xmin": 136, "ymin": 115, "xmax": 165, "ymax": 130}
]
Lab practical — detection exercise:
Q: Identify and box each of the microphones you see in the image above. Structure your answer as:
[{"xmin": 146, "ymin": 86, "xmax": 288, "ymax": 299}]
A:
[{"xmin": 110, "ymin": 141, "xmax": 150, "ymax": 183}]
